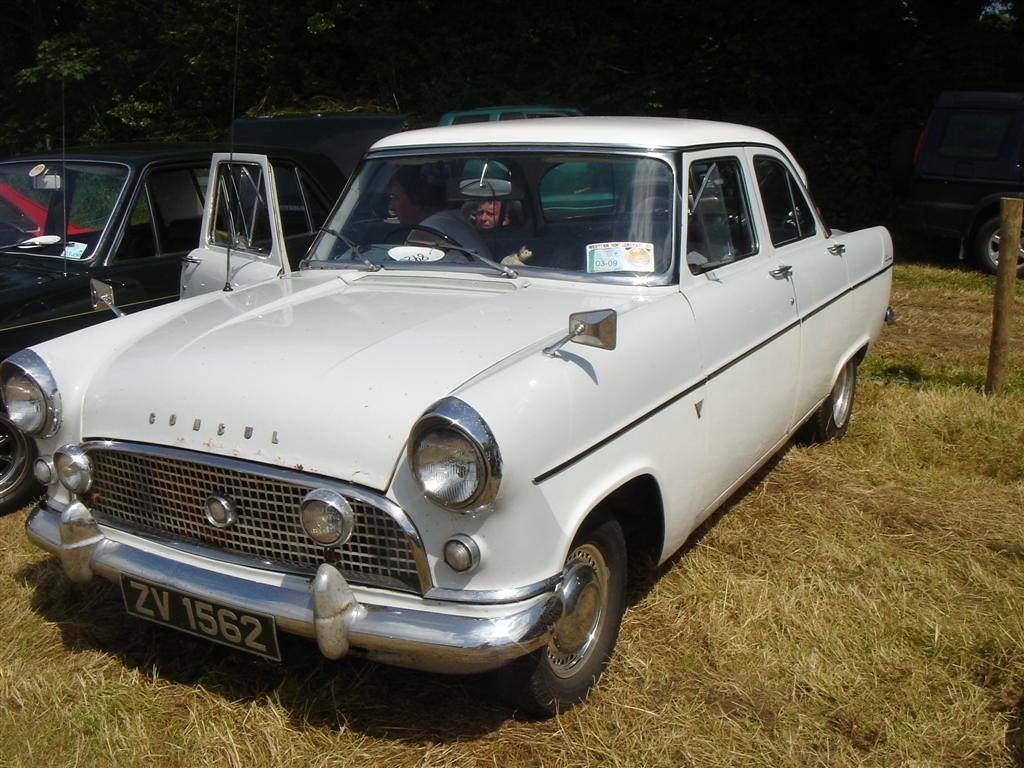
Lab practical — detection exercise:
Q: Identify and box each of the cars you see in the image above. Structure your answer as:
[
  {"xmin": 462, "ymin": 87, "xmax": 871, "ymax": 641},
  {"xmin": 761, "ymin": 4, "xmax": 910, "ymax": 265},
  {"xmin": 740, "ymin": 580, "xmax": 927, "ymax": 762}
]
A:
[
  {"xmin": 0, "ymin": 118, "xmax": 897, "ymax": 724},
  {"xmin": 0, "ymin": 141, "xmax": 406, "ymax": 518},
  {"xmin": 221, "ymin": 102, "xmax": 806, "ymax": 234}
]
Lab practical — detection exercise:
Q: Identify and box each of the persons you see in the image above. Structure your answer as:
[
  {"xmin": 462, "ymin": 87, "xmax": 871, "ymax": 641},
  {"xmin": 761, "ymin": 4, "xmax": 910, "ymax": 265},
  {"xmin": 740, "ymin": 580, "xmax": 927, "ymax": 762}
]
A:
[
  {"xmin": 389, "ymin": 159, "xmax": 493, "ymax": 264},
  {"xmin": 476, "ymin": 201, "xmax": 509, "ymax": 230}
]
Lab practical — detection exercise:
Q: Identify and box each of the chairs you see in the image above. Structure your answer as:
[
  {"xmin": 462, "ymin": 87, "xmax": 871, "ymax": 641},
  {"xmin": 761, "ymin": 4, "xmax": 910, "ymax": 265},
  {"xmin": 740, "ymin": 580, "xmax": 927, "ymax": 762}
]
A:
[
  {"xmin": 693, "ymin": 196, "xmax": 727, "ymax": 260},
  {"xmin": 126, "ymin": 217, "xmax": 201, "ymax": 257}
]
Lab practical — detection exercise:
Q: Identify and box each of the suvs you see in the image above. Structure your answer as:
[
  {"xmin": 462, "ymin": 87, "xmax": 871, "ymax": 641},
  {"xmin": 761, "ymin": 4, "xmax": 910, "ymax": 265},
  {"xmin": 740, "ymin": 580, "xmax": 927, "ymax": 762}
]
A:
[{"xmin": 894, "ymin": 91, "xmax": 1024, "ymax": 279}]
